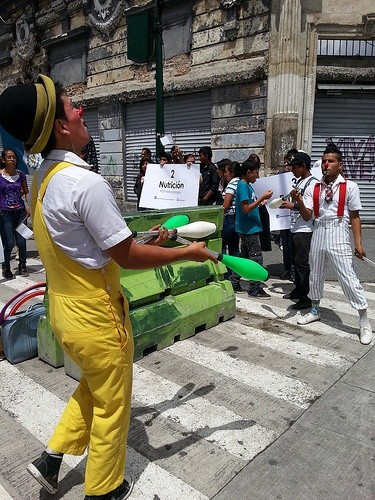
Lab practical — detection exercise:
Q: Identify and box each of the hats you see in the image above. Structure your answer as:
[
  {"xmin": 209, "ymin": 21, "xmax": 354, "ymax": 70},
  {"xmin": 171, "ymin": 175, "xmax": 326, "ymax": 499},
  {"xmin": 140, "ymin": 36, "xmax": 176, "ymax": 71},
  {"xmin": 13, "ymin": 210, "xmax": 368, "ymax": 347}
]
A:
[
  {"xmin": 0, "ymin": 74, "xmax": 56, "ymax": 154},
  {"xmin": 283, "ymin": 152, "xmax": 311, "ymax": 168}
]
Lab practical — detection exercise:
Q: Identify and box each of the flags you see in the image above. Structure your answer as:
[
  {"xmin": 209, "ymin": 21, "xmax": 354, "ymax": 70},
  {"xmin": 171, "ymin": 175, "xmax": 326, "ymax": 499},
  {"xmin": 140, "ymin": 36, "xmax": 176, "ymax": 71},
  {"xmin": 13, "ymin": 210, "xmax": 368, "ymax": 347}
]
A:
[{"xmin": 16, "ymin": 224, "xmax": 33, "ymax": 240}]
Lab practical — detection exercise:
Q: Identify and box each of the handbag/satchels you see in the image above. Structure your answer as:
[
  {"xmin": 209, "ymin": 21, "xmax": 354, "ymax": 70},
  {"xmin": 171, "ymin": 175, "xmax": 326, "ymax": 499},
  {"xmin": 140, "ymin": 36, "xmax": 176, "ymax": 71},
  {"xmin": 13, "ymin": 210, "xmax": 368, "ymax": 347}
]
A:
[{"xmin": 0, "ymin": 302, "xmax": 47, "ymax": 365}]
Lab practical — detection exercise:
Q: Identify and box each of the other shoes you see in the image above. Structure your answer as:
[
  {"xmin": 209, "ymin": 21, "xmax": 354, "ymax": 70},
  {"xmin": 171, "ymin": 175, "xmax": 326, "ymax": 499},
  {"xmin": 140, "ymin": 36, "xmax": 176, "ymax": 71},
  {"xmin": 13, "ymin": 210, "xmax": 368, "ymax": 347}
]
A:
[
  {"xmin": 18, "ymin": 263, "xmax": 29, "ymax": 276},
  {"xmin": 231, "ymin": 282, "xmax": 246, "ymax": 294},
  {"xmin": 291, "ymin": 299, "xmax": 312, "ymax": 310},
  {"xmin": 283, "ymin": 288, "xmax": 301, "ymax": 299},
  {"xmin": 360, "ymin": 323, "xmax": 372, "ymax": 344},
  {"xmin": 2, "ymin": 270, "xmax": 14, "ymax": 279},
  {"xmin": 297, "ymin": 312, "xmax": 320, "ymax": 325},
  {"xmin": 248, "ymin": 288, "xmax": 271, "ymax": 299}
]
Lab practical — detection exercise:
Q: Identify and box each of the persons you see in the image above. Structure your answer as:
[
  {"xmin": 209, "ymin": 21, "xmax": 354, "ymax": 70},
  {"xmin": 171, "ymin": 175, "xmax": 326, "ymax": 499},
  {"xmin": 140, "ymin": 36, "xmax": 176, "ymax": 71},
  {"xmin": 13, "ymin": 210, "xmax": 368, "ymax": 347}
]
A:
[
  {"xmin": 0, "ymin": 74, "xmax": 218, "ymax": 500},
  {"xmin": 133, "ymin": 146, "xmax": 217, "ymax": 212},
  {"xmin": 297, "ymin": 150, "xmax": 372, "ymax": 345},
  {"xmin": 0, "ymin": 148, "xmax": 31, "ymax": 283},
  {"xmin": 280, "ymin": 149, "xmax": 319, "ymax": 310},
  {"xmin": 314, "ymin": 143, "xmax": 339, "ymax": 169},
  {"xmin": 217, "ymin": 158, "xmax": 240, "ymax": 281},
  {"xmin": 230, "ymin": 153, "xmax": 274, "ymax": 300}
]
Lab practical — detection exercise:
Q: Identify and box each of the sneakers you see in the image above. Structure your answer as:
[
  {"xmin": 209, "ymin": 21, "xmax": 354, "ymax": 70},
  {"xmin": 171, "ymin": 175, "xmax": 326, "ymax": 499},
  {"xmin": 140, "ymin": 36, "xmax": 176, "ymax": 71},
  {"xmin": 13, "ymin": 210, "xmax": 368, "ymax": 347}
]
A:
[
  {"xmin": 26, "ymin": 449, "xmax": 62, "ymax": 495},
  {"xmin": 84, "ymin": 475, "xmax": 133, "ymax": 500}
]
